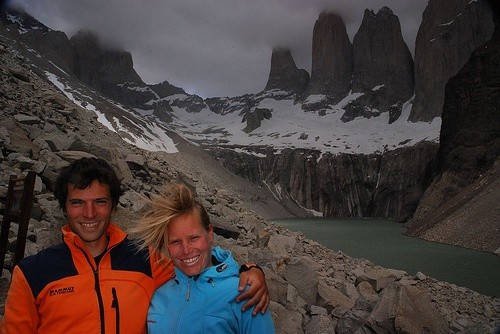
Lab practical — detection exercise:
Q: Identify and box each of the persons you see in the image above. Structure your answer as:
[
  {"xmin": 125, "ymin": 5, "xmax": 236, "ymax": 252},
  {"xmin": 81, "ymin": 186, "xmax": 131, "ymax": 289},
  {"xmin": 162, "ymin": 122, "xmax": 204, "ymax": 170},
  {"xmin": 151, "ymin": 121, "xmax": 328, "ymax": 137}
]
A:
[
  {"xmin": 0, "ymin": 157, "xmax": 270, "ymax": 334},
  {"xmin": 127, "ymin": 183, "xmax": 275, "ymax": 334}
]
